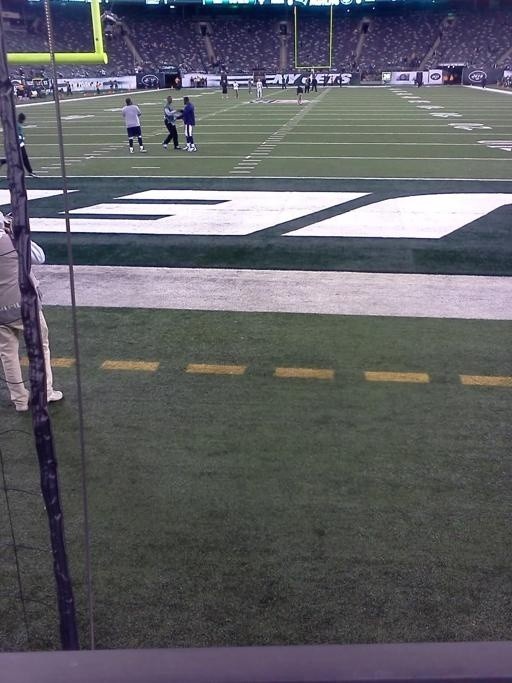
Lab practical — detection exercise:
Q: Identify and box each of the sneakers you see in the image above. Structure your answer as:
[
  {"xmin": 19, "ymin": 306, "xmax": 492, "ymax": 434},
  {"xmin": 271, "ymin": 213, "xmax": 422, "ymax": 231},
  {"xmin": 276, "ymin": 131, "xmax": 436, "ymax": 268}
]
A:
[
  {"xmin": 29, "ymin": 172, "xmax": 37, "ymax": 178},
  {"xmin": 130, "ymin": 142, "xmax": 196, "ymax": 154},
  {"xmin": 47, "ymin": 390, "xmax": 63, "ymax": 403},
  {"xmin": 14, "ymin": 402, "xmax": 29, "ymax": 412}
]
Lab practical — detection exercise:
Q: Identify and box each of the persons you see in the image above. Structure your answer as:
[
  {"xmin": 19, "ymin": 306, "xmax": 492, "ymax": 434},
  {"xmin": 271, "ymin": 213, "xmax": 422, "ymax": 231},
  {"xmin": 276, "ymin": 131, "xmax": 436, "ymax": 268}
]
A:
[
  {"xmin": 0, "ymin": 112, "xmax": 36, "ymax": 177},
  {"xmin": 121, "ymin": 98, "xmax": 147, "ymax": 153},
  {"xmin": 176, "ymin": 97, "xmax": 197, "ymax": 152},
  {"xmin": 0, "ymin": 213, "xmax": 65, "ymax": 412},
  {"xmin": 161, "ymin": 95, "xmax": 181, "ymax": 149}
]
